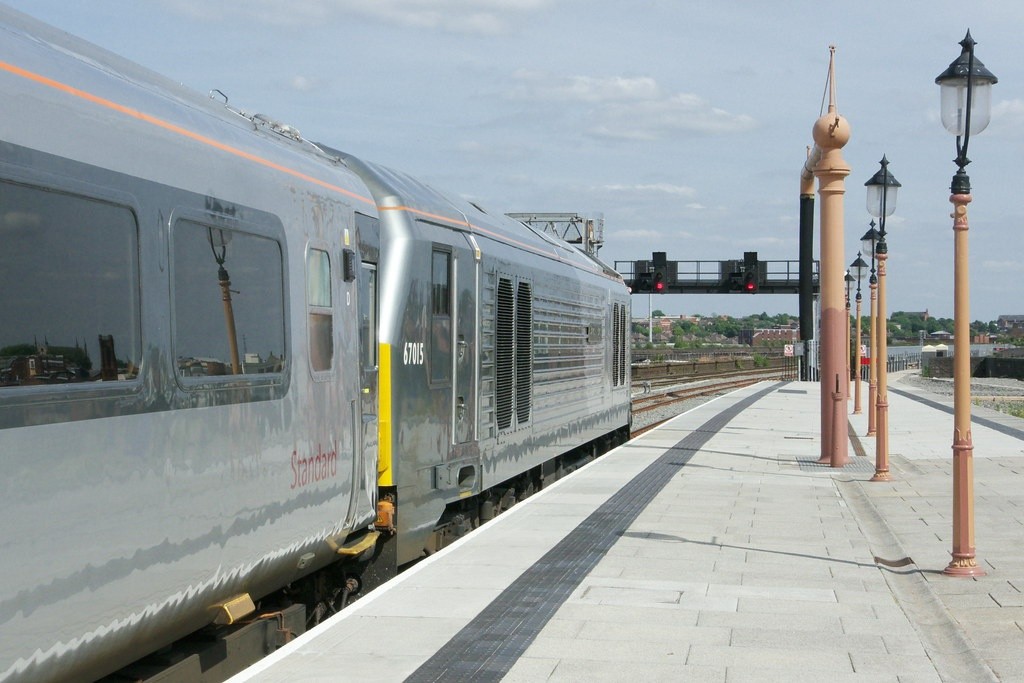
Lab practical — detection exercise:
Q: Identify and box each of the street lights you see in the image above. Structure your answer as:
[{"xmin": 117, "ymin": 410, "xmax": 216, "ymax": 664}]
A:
[
  {"xmin": 843, "ymin": 269, "xmax": 858, "ymax": 400},
  {"xmin": 849, "ymin": 249, "xmax": 868, "ymax": 415},
  {"xmin": 205, "ymin": 206, "xmax": 243, "ymax": 376},
  {"xmin": 860, "ymin": 217, "xmax": 882, "ymax": 439},
  {"xmin": 935, "ymin": 26, "xmax": 999, "ymax": 580},
  {"xmin": 863, "ymin": 151, "xmax": 903, "ymax": 483}
]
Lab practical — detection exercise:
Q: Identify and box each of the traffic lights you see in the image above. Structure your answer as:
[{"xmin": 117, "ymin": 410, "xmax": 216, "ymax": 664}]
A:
[
  {"xmin": 729, "ymin": 273, "xmax": 743, "ymax": 293},
  {"xmin": 639, "ymin": 272, "xmax": 652, "ymax": 292},
  {"xmin": 652, "ymin": 265, "xmax": 668, "ymax": 294},
  {"xmin": 744, "ymin": 269, "xmax": 758, "ymax": 294}
]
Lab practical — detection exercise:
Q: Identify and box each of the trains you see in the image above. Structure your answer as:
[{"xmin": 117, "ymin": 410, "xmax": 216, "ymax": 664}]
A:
[{"xmin": 0, "ymin": 2, "xmax": 636, "ymax": 683}]
